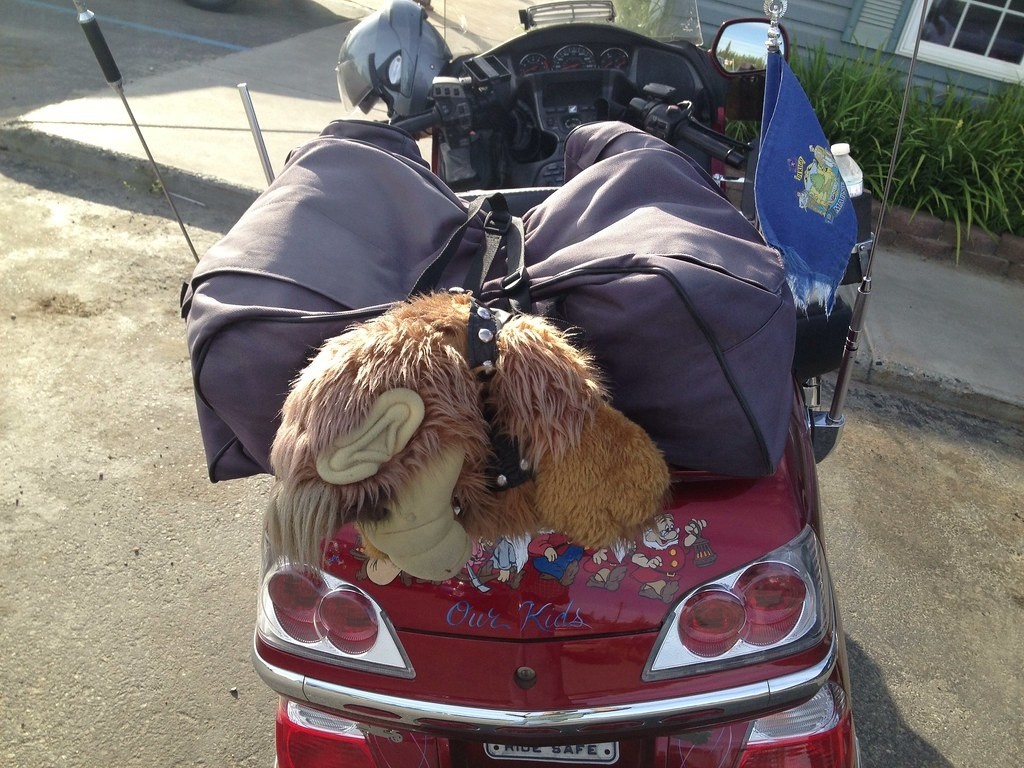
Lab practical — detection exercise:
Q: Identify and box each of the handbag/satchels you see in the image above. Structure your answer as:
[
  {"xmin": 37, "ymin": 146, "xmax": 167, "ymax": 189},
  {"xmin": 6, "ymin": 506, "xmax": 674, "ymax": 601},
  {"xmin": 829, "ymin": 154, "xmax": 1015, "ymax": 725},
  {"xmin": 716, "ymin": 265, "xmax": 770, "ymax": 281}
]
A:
[{"xmin": 181, "ymin": 121, "xmax": 795, "ymax": 482}]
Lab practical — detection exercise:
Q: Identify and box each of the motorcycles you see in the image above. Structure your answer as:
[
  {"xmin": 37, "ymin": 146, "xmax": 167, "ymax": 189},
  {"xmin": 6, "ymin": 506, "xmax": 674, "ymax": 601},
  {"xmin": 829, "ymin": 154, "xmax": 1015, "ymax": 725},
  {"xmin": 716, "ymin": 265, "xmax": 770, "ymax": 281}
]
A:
[{"xmin": 67, "ymin": 2, "xmax": 932, "ymax": 768}]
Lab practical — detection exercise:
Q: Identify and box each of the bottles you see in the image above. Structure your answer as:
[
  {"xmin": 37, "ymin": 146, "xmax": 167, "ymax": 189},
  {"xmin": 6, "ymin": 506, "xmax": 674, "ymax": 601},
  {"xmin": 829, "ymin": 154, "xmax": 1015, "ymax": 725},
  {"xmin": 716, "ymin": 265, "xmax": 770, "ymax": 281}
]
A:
[{"xmin": 831, "ymin": 142, "xmax": 863, "ymax": 197}]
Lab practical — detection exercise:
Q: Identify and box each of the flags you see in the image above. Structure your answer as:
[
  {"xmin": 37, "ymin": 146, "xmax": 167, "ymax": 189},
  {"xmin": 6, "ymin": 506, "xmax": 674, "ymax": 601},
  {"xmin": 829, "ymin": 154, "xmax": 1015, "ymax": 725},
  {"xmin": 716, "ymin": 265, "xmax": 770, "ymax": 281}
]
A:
[{"xmin": 753, "ymin": 50, "xmax": 857, "ymax": 320}]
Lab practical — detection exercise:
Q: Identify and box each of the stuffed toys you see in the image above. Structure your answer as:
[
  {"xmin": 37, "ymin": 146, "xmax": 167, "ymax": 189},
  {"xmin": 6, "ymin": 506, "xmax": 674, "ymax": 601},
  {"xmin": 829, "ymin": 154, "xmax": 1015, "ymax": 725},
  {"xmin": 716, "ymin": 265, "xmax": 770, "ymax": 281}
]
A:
[{"xmin": 262, "ymin": 294, "xmax": 672, "ymax": 586}]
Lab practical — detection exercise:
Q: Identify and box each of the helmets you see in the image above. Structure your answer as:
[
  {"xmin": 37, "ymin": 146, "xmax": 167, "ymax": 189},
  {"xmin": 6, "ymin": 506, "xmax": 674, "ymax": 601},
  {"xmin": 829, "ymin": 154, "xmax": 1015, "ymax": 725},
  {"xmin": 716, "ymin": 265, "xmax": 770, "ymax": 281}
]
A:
[{"xmin": 337, "ymin": 0, "xmax": 452, "ymax": 123}]
